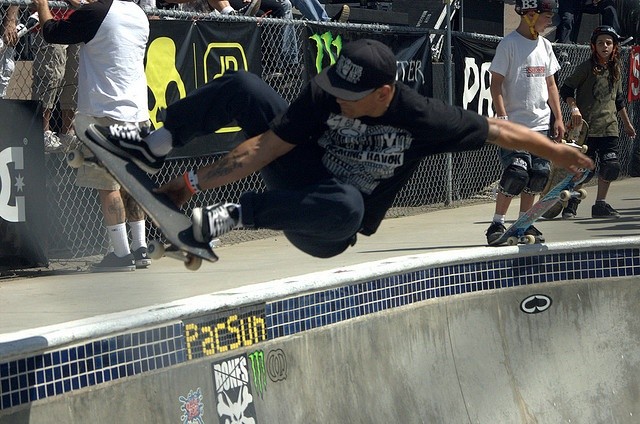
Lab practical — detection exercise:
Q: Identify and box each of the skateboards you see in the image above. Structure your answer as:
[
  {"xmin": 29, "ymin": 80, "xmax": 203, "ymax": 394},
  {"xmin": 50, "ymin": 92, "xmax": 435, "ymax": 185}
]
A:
[
  {"xmin": 538, "ymin": 117, "xmax": 592, "ymax": 218},
  {"xmin": 66, "ymin": 110, "xmax": 218, "ymax": 270},
  {"xmin": 489, "ymin": 168, "xmax": 590, "ymax": 245}
]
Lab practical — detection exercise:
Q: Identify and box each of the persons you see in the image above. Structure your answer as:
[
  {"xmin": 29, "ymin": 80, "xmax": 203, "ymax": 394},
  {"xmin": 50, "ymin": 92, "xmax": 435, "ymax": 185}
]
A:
[
  {"xmin": 87, "ymin": 38, "xmax": 594, "ymax": 258},
  {"xmin": 0, "ymin": 0, "xmax": 27, "ymax": 99},
  {"xmin": 562, "ymin": 25, "xmax": 635, "ymax": 219},
  {"xmin": 29, "ymin": 1, "xmax": 156, "ymax": 273},
  {"xmin": 206, "ymin": 0, "xmax": 260, "ymax": 19},
  {"xmin": 30, "ymin": 0, "xmax": 95, "ymax": 151},
  {"xmin": 261, "ymin": 1, "xmax": 351, "ymax": 84},
  {"xmin": 488, "ymin": 0, "xmax": 566, "ymax": 242}
]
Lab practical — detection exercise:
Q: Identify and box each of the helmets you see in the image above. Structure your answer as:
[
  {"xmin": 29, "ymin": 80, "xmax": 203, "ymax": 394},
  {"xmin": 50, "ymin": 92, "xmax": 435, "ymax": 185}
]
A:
[
  {"xmin": 591, "ymin": 25, "xmax": 619, "ymax": 41},
  {"xmin": 515, "ymin": 0, "xmax": 559, "ymax": 16}
]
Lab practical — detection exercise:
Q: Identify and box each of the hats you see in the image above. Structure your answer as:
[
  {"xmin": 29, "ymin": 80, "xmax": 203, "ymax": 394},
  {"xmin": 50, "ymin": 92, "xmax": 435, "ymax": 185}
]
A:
[{"xmin": 314, "ymin": 39, "xmax": 396, "ymax": 102}]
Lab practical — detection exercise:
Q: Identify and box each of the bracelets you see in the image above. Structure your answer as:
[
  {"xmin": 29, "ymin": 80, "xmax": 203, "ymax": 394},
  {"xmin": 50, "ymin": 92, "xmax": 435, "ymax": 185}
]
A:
[
  {"xmin": 497, "ymin": 114, "xmax": 510, "ymax": 122},
  {"xmin": 184, "ymin": 168, "xmax": 202, "ymax": 193},
  {"xmin": 570, "ymin": 106, "xmax": 579, "ymax": 113}
]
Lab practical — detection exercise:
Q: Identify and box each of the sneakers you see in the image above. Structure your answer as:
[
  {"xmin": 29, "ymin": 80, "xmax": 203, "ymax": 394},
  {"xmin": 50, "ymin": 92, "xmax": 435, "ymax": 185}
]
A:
[
  {"xmin": 90, "ymin": 250, "xmax": 136, "ymax": 272},
  {"xmin": 43, "ymin": 130, "xmax": 66, "ymax": 153},
  {"xmin": 191, "ymin": 200, "xmax": 239, "ymax": 243},
  {"xmin": 332, "ymin": 3, "xmax": 351, "ymax": 22},
  {"xmin": 58, "ymin": 127, "xmax": 77, "ymax": 149},
  {"xmin": 563, "ymin": 197, "xmax": 581, "ymax": 220},
  {"xmin": 526, "ymin": 223, "xmax": 545, "ymax": 244},
  {"xmin": 87, "ymin": 122, "xmax": 167, "ymax": 174},
  {"xmin": 591, "ymin": 200, "xmax": 621, "ymax": 218},
  {"xmin": 486, "ymin": 222, "xmax": 506, "ymax": 243},
  {"xmin": 131, "ymin": 246, "xmax": 151, "ymax": 268},
  {"xmin": 233, "ymin": 0, "xmax": 261, "ymax": 18},
  {"xmin": 619, "ymin": 36, "xmax": 634, "ymax": 47}
]
